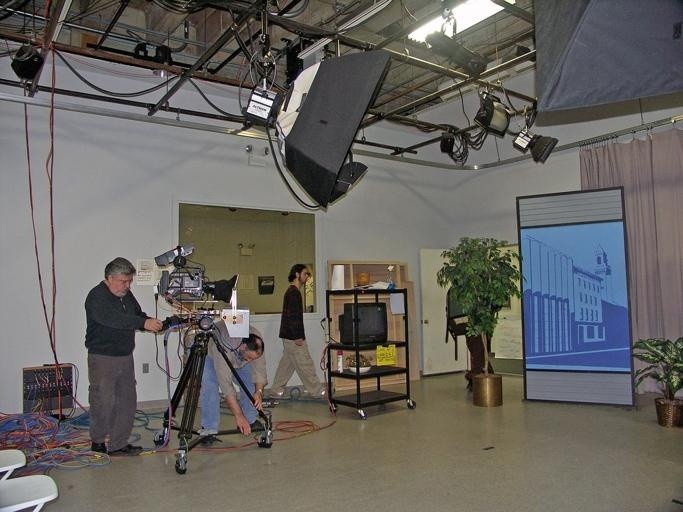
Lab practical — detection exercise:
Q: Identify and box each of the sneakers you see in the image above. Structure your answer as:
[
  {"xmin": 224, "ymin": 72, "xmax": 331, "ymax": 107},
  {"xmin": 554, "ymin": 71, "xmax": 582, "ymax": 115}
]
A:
[
  {"xmin": 269, "ymin": 391, "xmax": 287, "ymax": 398},
  {"xmin": 237, "ymin": 420, "xmax": 265, "ymax": 433},
  {"xmin": 201, "ymin": 435, "xmax": 215, "ymax": 447},
  {"xmin": 313, "ymin": 387, "xmax": 335, "ymax": 399}
]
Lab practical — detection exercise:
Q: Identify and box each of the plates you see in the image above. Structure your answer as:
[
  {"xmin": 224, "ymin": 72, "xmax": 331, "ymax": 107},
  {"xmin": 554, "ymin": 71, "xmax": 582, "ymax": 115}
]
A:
[{"xmin": 349, "ymin": 366, "xmax": 370, "ymax": 374}]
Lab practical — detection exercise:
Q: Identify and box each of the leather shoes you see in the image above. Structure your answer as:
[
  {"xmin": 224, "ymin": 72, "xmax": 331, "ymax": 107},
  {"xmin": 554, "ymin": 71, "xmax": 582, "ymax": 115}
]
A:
[
  {"xmin": 107, "ymin": 444, "xmax": 142, "ymax": 455},
  {"xmin": 91, "ymin": 442, "xmax": 107, "ymax": 453}
]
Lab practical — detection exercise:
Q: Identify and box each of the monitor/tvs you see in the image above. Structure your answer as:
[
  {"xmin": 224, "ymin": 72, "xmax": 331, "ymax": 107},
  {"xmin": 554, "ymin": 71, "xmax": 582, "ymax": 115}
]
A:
[{"xmin": 338, "ymin": 303, "xmax": 388, "ymax": 346}]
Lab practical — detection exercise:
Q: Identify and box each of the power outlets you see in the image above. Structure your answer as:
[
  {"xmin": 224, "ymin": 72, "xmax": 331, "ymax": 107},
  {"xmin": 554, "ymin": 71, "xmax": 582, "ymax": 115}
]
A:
[{"xmin": 143, "ymin": 363, "xmax": 149, "ymax": 374}]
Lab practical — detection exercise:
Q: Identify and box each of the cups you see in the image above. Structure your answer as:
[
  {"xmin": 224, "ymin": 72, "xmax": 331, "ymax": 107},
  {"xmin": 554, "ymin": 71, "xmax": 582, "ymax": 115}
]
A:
[{"xmin": 356, "ymin": 272, "xmax": 371, "ymax": 286}]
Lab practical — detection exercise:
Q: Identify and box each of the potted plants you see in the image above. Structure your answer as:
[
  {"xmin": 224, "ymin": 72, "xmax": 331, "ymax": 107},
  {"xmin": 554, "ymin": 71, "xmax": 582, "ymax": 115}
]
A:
[
  {"xmin": 437, "ymin": 235, "xmax": 525, "ymax": 407},
  {"xmin": 631, "ymin": 338, "xmax": 683, "ymax": 427}
]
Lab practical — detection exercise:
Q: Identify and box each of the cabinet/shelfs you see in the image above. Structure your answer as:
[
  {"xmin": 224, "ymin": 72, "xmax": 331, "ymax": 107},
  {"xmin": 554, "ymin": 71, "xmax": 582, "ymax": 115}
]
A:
[
  {"xmin": 327, "ymin": 260, "xmax": 420, "ymax": 397},
  {"xmin": 325, "ymin": 288, "xmax": 417, "ymax": 420}
]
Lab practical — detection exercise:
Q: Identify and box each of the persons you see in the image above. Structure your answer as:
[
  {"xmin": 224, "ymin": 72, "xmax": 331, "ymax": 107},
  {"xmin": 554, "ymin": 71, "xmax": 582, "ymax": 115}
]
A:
[
  {"xmin": 465, "ymin": 319, "xmax": 494, "ymax": 392},
  {"xmin": 85, "ymin": 257, "xmax": 164, "ymax": 455},
  {"xmin": 197, "ymin": 319, "xmax": 268, "ymax": 442},
  {"xmin": 269, "ymin": 263, "xmax": 323, "ymax": 398}
]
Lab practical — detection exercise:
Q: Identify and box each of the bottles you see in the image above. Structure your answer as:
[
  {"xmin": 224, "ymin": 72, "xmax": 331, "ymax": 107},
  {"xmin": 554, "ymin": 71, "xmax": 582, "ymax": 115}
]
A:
[
  {"xmin": 338, "ymin": 349, "xmax": 342, "ymax": 373},
  {"xmin": 386, "ymin": 270, "xmax": 395, "ymax": 289}
]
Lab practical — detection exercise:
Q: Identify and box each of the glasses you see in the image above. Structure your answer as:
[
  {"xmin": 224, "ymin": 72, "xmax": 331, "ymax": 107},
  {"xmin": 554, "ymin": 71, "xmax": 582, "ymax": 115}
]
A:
[
  {"xmin": 113, "ymin": 279, "xmax": 133, "ymax": 283},
  {"xmin": 301, "ymin": 272, "xmax": 311, "ymax": 277}
]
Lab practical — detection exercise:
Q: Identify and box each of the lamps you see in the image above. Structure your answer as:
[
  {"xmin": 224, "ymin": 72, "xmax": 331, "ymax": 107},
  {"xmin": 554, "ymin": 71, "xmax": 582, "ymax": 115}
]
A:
[
  {"xmin": 425, "ymin": 31, "xmax": 490, "ymax": 81},
  {"xmin": 513, "ymin": 127, "xmax": 559, "ymax": 164},
  {"xmin": 474, "ymin": 97, "xmax": 511, "ymax": 138},
  {"xmin": 327, "ymin": 160, "xmax": 368, "ymax": 204},
  {"xmin": 408, "ymin": 0, "xmax": 516, "ymax": 49}
]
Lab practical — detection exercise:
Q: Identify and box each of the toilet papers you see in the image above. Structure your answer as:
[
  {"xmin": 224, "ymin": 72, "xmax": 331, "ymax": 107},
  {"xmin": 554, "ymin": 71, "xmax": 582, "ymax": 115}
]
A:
[{"xmin": 331, "ymin": 265, "xmax": 344, "ymax": 291}]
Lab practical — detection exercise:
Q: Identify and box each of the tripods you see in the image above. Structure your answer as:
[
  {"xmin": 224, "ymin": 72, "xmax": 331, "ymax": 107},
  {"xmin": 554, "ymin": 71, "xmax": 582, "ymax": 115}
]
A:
[{"xmin": 153, "ymin": 329, "xmax": 274, "ymax": 474}]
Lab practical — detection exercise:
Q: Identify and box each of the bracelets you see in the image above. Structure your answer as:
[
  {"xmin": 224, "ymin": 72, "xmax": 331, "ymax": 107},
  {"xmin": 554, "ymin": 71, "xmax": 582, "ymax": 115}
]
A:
[{"xmin": 255, "ymin": 388, "xmax": 263, "ymax": 397}]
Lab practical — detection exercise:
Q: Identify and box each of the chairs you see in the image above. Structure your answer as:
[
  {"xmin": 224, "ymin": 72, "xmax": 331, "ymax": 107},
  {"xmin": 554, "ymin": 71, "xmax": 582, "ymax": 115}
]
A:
[
  {"xmin": 0, "ymin": 449, "xmax": 26, "ymax": 478},
  {"xmin": 1, "ymin": 475, "xmax": 58, "ymax": 512}
]
